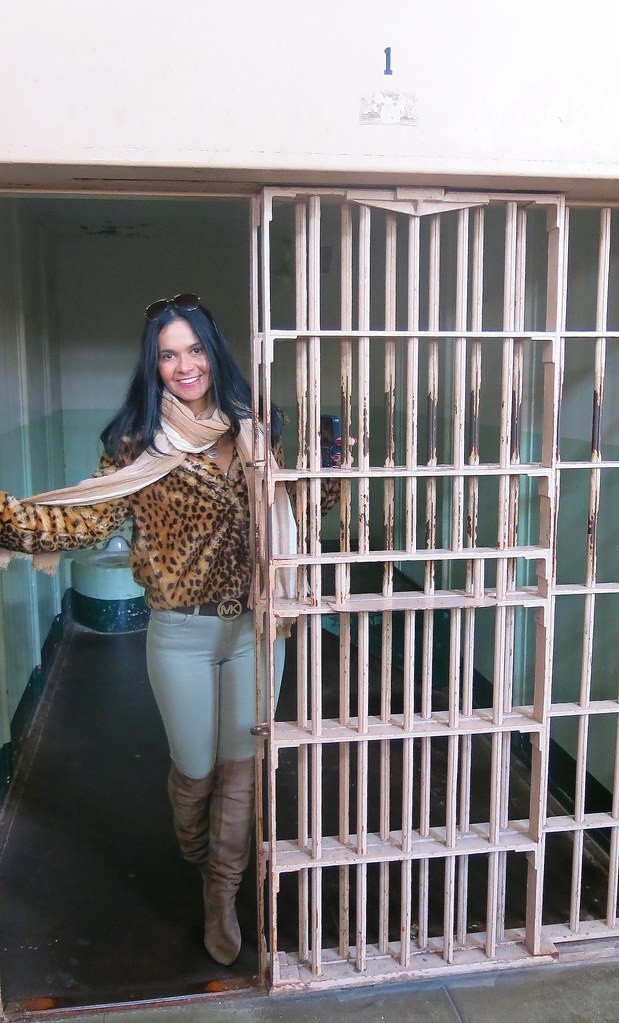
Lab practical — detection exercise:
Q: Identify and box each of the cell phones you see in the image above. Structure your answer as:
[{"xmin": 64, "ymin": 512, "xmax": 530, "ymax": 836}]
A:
[{"xmin": 319, "ymin": 415, "xmax": 342, "ymax": 468}]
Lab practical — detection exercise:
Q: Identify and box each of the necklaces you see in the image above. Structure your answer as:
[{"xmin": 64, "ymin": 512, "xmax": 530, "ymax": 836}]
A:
[{"xmin": 202, "ymin": 439, "xmax": 221, "ymax": 459}]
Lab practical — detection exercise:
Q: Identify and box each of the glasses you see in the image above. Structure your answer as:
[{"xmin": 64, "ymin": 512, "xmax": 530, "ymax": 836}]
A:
[{"xmin": 144, "ymin": 294, "xmax": 201, "ymax": 321}]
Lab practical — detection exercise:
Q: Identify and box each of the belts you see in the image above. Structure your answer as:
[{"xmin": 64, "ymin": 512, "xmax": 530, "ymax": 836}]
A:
[{"xmin": 170, "ymin": 595, "xmax": 251, "ymax": 621}]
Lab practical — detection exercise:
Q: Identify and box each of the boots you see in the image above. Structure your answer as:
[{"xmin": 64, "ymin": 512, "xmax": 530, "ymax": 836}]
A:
[
  {"xmin": 203, "ymin": 755, "xmax": 255, "ymax": 966},
  {"xmin": 167, "ymin": 759, "xmax": 210, "ymax": 867}
]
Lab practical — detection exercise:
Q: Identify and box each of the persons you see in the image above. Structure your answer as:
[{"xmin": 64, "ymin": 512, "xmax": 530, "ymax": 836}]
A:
[{"xmin": 0, "ymin": 293, "xmax": 355, "ymax": 964}]
